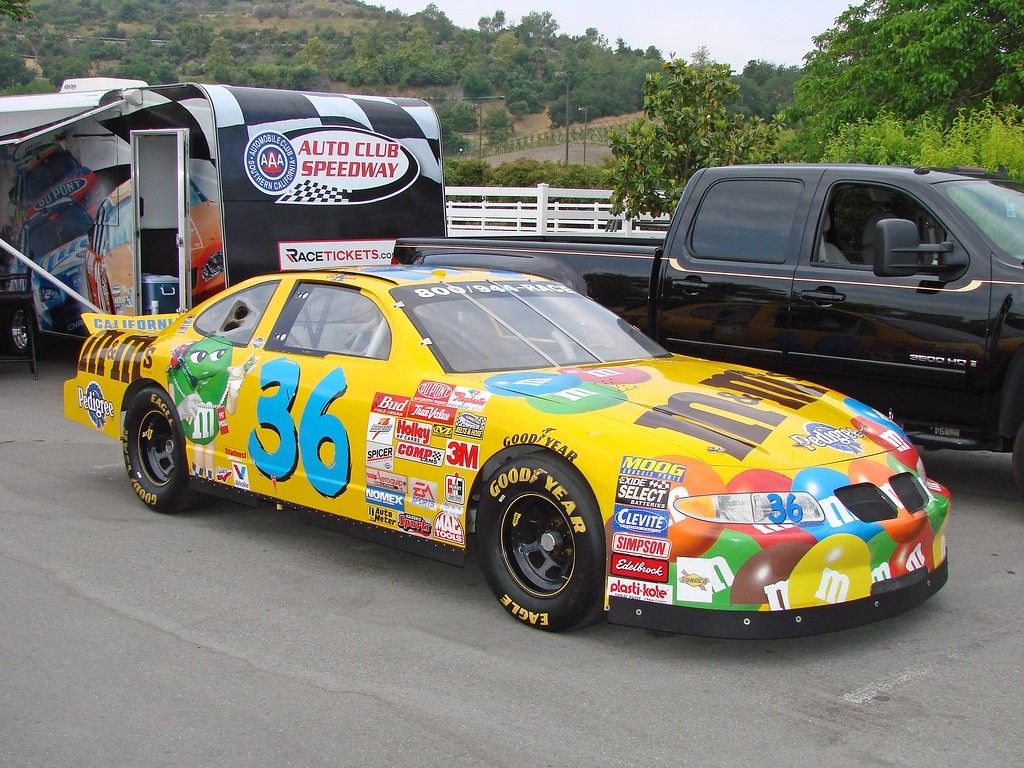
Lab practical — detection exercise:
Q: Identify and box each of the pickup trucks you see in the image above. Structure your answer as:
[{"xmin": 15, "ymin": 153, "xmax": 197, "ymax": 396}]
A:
[{"xmin": 393, "ymin": 162, "xmax": 1024, "ymax": 503}]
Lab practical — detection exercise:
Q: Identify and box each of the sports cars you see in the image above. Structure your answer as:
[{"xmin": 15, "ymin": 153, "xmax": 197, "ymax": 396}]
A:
[{"xmin": 61, "ymin": 263, "xmax": 954, "ymax": 635}]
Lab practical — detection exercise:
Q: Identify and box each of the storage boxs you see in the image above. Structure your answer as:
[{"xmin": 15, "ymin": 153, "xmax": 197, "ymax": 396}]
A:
[{"xmin": 141, "ymin": 273, "xmax": 179, "ymax": 315}]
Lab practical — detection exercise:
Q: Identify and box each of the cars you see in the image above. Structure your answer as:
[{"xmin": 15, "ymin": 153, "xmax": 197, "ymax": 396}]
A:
[
  {"xmin": 11, "ymin": 140, "xmax": 105, "ymax": 222},
  {"xmin": 9, "ymin": 195, "xmax": 107, "ymax": 341}
]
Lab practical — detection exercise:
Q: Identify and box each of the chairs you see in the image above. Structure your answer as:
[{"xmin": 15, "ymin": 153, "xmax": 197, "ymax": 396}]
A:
[
  {"xmin": 861, "ymin": 212, "xmax": 923, "ymax": 264},
  {"xmin": 786, "ymin": 203, "xmax": 851, "ymax": 265}
]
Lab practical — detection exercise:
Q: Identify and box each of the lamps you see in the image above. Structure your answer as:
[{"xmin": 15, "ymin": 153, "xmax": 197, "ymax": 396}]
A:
[{"xmin": 123, "ymin": 89, "xmax": 144, "ymax": 106}]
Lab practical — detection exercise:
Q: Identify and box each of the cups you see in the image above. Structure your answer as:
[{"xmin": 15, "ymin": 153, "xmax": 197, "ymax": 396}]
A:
[{"xmin": 151, "ymin": 301, "xmax": 158, "ymax": 315}]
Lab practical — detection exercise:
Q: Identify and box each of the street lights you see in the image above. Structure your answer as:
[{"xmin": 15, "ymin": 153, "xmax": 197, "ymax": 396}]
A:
[
  {"xmin": 554, "ymin": 72, "xmax": 570, "ymax": 160},
  {"xmin": 579, "ymin": 106, "xmax": 588, "ymax": 162}
]
